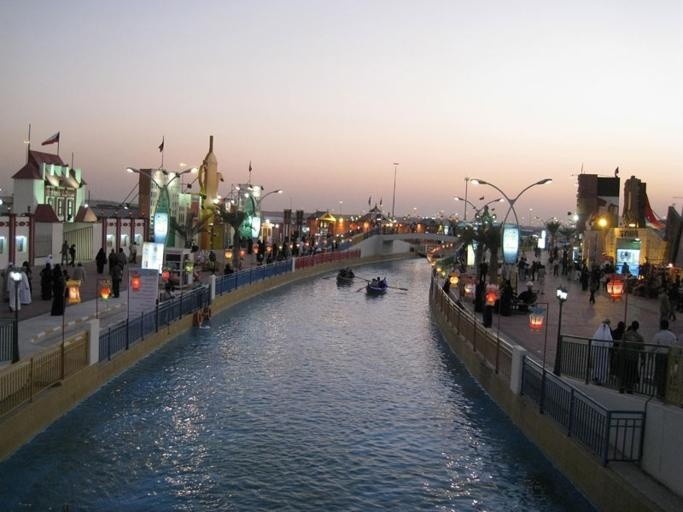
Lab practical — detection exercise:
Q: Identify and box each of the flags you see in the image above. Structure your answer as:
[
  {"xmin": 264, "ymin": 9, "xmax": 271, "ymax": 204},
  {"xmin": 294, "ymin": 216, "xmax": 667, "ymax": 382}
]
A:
[
  {"xmin": 248, "ymin": 162, "xmax": 252, "ymax": 171},
  {"xmin": 41, "ymin": 132, "xmax": 59, "ymax": 146},
  {"xmin": 158, "ymin": 140, "xmax": 164, "ymax": 152},
  {"xmin": 645, "ymin": 194, "xmax": 666, "ymax": 231}
]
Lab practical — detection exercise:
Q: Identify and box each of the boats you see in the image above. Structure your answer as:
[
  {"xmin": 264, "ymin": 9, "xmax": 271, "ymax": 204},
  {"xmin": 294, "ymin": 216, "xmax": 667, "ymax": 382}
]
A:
[
  {"xmin": 366, "ymin": 276, "xmax": 388, "ymax": 295},
  {"xmin": 336, "ymin": 267, "xmax": 355, "ymax": 288}
]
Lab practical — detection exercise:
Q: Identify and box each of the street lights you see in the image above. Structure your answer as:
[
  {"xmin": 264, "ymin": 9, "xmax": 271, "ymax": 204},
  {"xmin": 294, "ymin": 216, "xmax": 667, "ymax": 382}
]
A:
[
  {"xmin": 9, "ymin": 267, "xmax": 26, "ymax": 364},
  {"xmin": 212, "ymin": 187, "xmax": 283, "ymax": 278},
  {"xmin": 125, "ymin": 167, "xmax": 199, "ymax": 270},
  {"xmin": 391, "ymin": 162, "xmax": 400, "ymax": 218},
  {"xmin": 454, "ymin": 177, "xmax": 568, "ymax": 374},
  {"xmin": 339, "ymin": 200, "xmax": 344, "ymax": 216}
]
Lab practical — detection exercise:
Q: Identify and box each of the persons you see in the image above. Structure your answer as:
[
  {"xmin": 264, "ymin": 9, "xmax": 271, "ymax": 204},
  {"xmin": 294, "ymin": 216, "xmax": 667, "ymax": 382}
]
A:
[
  {"xmin": 349, "ymin": 270, "xmax": 354, "ymax": 275},
  {"xmin": 376, "ymin": 276, "xmax": 380, "ymax": 286},
  {"xmin": 51, "ymin": 275, "xmax": 66, "ymax": 315},
  {"xmin": 40, "ymin": 262, "xmax": 86, "ymax": 300},
  {"xmin": 472, "ymin": 244, "xmax": 683, "ymax": 401},
  {"xmin": 346, "ymin": 266, "xmax": 350, "ymax": 272},
  {"xmin": 7, "ymin": 261, "xmax": 34, "ymax": 312},
  {"xmin": 240, "ymin": 232, "xmax": 339, "ymax": 266},
  {"xmin": 61, "ymin": 240, "xmax": 76, "ymax": 267},
  {"xmin": 164, "ymin": 277, "xmax": 176, "ymax": 322},
  {"xmin": 191, "ymin": 240, "xmax": 234, "ymax": 293},
  {"xmin": 340, "ymin": 270, "xmax": 346, "ymax": 277},
  {"xmin": 371, "ymin": 278, "xmax": 377, "ymax": 286},
  {"xmin": 95, "ymin": 241, "xmax": 137, "ymax": 298}
]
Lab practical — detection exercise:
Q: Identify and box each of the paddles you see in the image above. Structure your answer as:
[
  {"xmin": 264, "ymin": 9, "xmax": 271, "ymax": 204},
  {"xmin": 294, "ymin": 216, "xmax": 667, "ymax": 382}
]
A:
[
  {"xmin": 322, "ymin": 275, "xmax": 337, "ymax": 279},
  {"xmin": 356, "ymin": 285, "xmax": 370, "ymax": 292},
  {"xmin": 356, "ymin": 276, "xmax": 371, "ymax": 282},
  {"xmin": 386, "ymin": 286, "xmax": 408, "ymax": 291}
]
